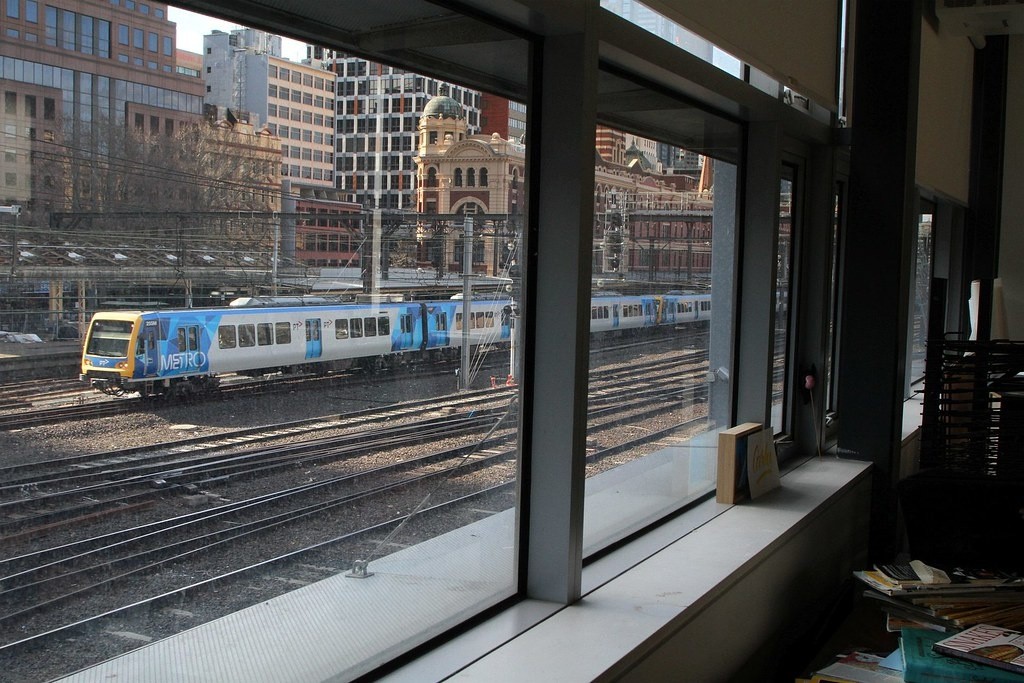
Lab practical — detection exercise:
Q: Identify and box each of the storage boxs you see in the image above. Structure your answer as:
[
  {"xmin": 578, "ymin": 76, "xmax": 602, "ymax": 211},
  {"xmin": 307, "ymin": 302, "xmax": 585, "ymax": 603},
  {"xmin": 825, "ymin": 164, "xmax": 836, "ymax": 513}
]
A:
[{"xmin": 717, "ymin": 422, "xmax": 763, "ymax": 504}]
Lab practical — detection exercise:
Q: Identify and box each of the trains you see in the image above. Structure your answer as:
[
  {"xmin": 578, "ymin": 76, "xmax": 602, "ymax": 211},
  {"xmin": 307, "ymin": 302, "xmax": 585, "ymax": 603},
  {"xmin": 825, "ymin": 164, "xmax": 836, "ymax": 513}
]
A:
[{"xmin": 79, "ymin": 290, "xmax": 789, "ymax": 401}]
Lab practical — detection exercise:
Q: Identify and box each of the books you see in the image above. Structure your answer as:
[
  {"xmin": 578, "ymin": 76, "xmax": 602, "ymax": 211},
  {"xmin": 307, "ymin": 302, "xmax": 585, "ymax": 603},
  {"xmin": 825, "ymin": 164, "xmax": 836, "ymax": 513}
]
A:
[{"xmin": 793, "ymin": 561, "xmax": 1024, "ymax": 683}]
